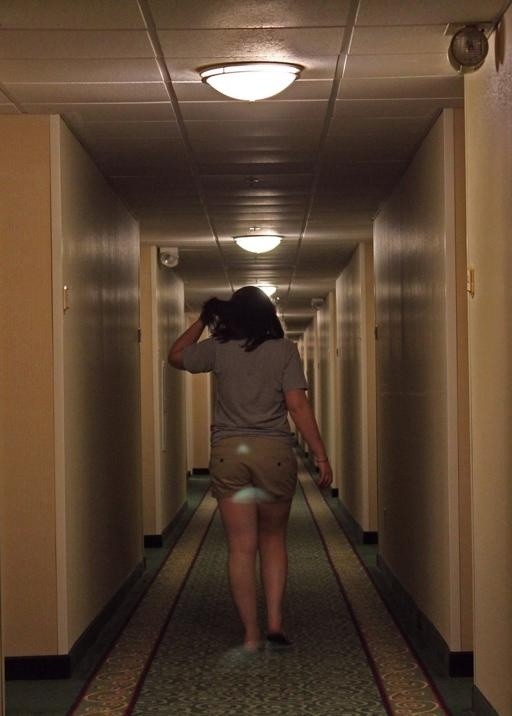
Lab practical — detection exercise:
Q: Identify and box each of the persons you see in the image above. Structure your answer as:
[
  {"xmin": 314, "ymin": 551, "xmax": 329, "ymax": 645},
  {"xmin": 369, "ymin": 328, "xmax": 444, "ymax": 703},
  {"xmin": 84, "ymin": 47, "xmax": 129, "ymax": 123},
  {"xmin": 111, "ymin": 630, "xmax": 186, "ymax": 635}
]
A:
[{"xmin": 168, "ymin": 285, "xmax": 335, "ymax": 658}]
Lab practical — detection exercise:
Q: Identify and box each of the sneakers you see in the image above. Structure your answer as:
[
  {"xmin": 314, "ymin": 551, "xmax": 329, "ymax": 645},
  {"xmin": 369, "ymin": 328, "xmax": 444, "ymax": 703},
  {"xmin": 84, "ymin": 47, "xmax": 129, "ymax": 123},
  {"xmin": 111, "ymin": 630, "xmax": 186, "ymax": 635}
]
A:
[{"xmin": 263, "ymin": 631, "xmax": 293, "ymax": 649}]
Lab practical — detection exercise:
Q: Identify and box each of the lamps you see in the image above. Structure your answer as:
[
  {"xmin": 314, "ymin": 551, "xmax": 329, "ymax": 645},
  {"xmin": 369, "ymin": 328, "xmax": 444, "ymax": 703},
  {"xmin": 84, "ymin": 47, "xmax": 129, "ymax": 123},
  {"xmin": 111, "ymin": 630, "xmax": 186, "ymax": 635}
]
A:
[
  {"xmin": 198, "ymin": 63, "xmax": 304, "ymax": 102},
  {"xmin": 234, "ymin": 236, "xmax": 284, "ymax": 254}
]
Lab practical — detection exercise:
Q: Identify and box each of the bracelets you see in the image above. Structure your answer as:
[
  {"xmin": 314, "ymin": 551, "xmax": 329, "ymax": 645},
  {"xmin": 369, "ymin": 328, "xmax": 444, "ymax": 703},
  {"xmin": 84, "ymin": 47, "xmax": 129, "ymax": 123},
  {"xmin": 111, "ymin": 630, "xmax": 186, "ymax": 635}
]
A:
[{"xmin": 311, "ymin": 454, "xmax": 329, "ymax": 464}]
